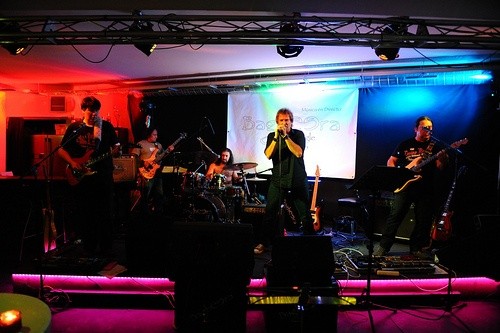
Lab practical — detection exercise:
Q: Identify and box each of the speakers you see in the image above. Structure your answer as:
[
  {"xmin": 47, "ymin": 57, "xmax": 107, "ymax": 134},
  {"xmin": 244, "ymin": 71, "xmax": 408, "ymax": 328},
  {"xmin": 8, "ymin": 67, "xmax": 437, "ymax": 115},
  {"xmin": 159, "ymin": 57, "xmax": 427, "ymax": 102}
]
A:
[
  {"xmin": 266, "ymin": 232, "xmax": 335, "ymax": 286},
  {"xmin": 170, "ymin": 219, "xmax": 255, "ymax": 286}
]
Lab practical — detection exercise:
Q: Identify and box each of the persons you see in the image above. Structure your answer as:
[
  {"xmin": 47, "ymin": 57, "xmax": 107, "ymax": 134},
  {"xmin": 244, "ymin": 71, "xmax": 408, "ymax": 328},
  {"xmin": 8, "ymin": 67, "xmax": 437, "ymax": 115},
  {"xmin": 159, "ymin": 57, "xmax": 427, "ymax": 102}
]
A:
[
  {"xmin": 253, "ymin": 109, "xmax": 316, "ymax": 254},
  {"xmin": 206, "ymin": 148, "xmax": 243, "ymax": 187},
  {"xmin": 57, "ymin": 96, "xmax": 121, "ymax": 258},
  {"xmin": 375, "ymin": 116, "xmax": 449, "ymax": 257},
  {"xmin": 131, "ymin": 127, "xmax": 174, "ymax": 199}
]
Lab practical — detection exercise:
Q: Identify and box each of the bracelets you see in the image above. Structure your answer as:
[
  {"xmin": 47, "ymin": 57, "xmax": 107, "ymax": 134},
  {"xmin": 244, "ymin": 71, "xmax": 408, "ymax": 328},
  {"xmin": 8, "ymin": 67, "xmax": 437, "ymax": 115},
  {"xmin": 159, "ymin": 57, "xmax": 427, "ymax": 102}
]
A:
[
  {"xmin": 272, "ymin": 138, "xmax": 278, "ymax": 142},
  {"xmin": 283, "ymin": 134, "xmax": 289, "ymax": 139}
]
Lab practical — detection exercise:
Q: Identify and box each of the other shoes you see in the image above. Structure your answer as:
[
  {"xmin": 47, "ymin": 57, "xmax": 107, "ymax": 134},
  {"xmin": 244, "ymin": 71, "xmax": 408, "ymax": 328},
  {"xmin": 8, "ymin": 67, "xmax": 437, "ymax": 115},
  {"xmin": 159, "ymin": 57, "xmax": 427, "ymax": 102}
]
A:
[
  {"xmin": 409, "ymin": 249, "xmax": 423, "ymax": 260},
  {"xmin": 76, "ymin": 243, "xmax": 92, "ymax": 257},
  {"xmin": 373, "ymin": 247, "xmax": 389, "ymax": 256},
  {"xmin": 100, "ymin": 247, "xmax": 112, "ymax": 258}
]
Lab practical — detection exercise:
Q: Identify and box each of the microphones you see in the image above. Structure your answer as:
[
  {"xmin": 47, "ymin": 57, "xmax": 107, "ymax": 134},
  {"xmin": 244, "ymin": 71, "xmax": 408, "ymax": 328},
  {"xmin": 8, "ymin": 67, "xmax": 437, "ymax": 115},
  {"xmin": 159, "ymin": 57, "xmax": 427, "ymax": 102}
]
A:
[{"xmin": 211, "ymin": 150, "xmax": 221, "ymax": 159}]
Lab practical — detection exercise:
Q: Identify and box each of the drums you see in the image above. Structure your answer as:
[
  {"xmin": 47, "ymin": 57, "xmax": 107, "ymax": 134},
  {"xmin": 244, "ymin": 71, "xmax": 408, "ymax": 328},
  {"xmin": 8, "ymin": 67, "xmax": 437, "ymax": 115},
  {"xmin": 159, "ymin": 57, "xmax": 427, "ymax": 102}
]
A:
[
  {"xmin": 222, "ymin": 186, "xmax": 242, "ymax": 197},
  {"xmin": 207, "ymin": 173, "xmax": 225, "ymax": 192},
  {"xmin": 183, "ymin": 172, "xmax": 206, "ymax": 192},
  {"xmin": 180, "ymin": 195, "xmax": 225, "ymax": 224}
]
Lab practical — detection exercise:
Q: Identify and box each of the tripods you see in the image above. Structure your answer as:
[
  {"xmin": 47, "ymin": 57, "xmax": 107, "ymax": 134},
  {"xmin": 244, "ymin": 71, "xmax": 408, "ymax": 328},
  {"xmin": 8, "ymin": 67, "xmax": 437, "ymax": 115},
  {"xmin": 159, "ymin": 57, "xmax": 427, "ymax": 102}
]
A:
[
  {"xmin": 408, "ymin": 257, "xmax": 471, "ymax": 333},
  {"xmin": 335, "ymin": 164, "xmax": 404, "ymax": 331}
]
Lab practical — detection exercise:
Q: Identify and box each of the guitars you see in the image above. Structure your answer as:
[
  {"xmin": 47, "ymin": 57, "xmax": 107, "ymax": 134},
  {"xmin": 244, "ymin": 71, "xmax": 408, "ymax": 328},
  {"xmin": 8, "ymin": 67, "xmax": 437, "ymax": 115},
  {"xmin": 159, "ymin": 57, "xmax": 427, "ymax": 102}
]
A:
[
  {"xmin": 393, "ymin": 137, "xmax": 468, "ymax": 193},
  {"xmin": 138, "ymin": 132, "xmax": 188, "ymax": 179},
  {"xmin": 64, "ymin": 143, "xmax": 123, "ymax": 185},
  {"xmin": 310, "ymin": 169, "xmax": 320, "ymax": 231},
  {"xmin": 429, "ymin": 166, "xmax": 466, "ymax": 240}
]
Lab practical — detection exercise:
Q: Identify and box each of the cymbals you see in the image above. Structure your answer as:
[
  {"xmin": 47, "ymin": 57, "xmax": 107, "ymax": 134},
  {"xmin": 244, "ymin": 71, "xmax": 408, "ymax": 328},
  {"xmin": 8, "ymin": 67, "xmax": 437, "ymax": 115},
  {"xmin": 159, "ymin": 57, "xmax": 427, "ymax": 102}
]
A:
[
  {"xmin": 223, "ymin": 163, "xmax": 257, "ymax": 171},
  {"xmin": 246, "ymin": 177, "xmax": 268, "ymax": 182}
]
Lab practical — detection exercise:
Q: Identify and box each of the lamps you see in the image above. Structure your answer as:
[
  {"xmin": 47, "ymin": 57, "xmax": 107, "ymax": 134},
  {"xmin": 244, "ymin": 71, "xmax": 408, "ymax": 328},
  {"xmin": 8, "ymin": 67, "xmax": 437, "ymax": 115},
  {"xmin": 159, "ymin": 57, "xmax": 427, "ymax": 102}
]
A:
[
  {"xmin": 374, "ymin": 25, "xmax": 400, "ymax": 62},
  {"xmin": 275, "ymin": 19, "xmax": 304, "ymax": 58},
  {"xmin": 0, "ymin": 21, "xmax": 30, "ymax": 55},
  {"xmin": 129, "ymin": 21, "xmax": 157, "ymax": 56}
]
